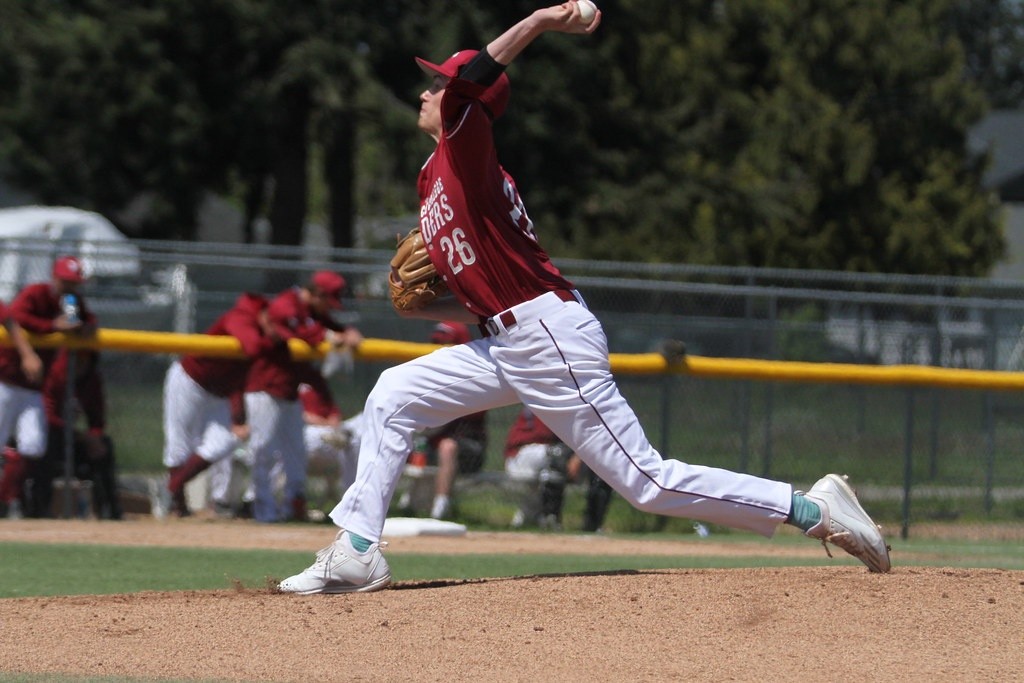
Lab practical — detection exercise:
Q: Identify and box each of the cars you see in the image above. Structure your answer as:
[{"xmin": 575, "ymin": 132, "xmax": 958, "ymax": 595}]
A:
[{"xmin": 83, "ymin": 250, "xmax": 472, "ymax": 380}]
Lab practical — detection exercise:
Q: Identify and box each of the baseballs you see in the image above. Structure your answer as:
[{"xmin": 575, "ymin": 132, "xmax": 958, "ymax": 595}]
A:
[{"xmin": 575, "ymin": 0, "xmax": 598, "ymax": 25}]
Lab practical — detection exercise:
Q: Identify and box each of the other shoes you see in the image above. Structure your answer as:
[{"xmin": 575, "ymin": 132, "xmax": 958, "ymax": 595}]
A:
[
  {"xmin": 396, "ymin": 492, "xmax": 415, "ymax": 517},
  {"xmin": 430, "ymin": 493, "xmax": 450, "ymax": 519}
]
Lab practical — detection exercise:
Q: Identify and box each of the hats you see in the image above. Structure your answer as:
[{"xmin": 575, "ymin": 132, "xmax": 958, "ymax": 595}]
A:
[
  {"xmin": 310, "ymin": 271, "xmax": 347, "ymax": 311},
  {"xmin": 430, "ymin": 320, "xmax": 470, "ymax": 345},
  {"xmin": 415, "ymin": 49, "xmax": 511, "ymax": 121},
  {"xmin": 53, "ymin": 256, "xmax": 87, "ymax": 282}
]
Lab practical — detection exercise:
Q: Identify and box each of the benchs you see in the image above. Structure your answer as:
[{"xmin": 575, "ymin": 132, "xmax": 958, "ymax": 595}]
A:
[{"xmin": 396, "ymin": 463, "xmax": 588, "ymax": 532}]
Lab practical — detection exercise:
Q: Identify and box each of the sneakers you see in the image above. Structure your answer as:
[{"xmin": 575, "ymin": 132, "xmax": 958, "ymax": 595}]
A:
[
  {"xmin": 794, "ymin": 473, "xmax": 891, "ymax": 573},
  {"xmin": 146, "ymin": 473, "xmax": 192, "ymax": 525},
  {"xmin": 277, "ymin": 529, "xmax": 392, "ymax": 596}
]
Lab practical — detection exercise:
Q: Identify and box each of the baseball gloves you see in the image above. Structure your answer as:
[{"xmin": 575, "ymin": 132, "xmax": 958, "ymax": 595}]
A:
[{"xmin": 386, "ymin": 226, "xmax": 486, "ymax": 325}]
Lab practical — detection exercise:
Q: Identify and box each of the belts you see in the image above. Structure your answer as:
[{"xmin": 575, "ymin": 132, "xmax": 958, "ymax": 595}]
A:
[{"xmin": 478, "ymin": 288, "xmax": 579, "ymax": 337}]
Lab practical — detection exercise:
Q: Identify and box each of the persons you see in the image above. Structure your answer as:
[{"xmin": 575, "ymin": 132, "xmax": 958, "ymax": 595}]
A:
[
  {"xmin": 396, "ymin": 322, "xmax": 488, "ymax": 521},
  {"xmin": 274, "ymin": 0, "xmax": 893, "ymax": 598},
  {"xmin": 0, "ymin": 257, "xmax": 124, "ymax": 522},
  {"xmin": 147, "ymin": 267, "xmax": 365, "ymax": 522},
  {"xmin": 503, "ymin": 404, "xmax": 584, "ymax": 531}
]
